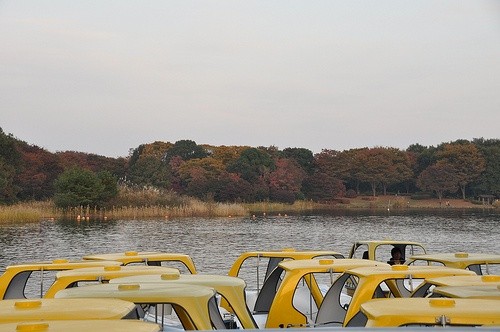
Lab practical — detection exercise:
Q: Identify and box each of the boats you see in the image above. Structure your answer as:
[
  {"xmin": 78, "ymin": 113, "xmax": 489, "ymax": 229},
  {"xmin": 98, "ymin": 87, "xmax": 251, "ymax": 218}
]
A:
[{"xmin": 0, "ymin": 240, "xmax": 500, "ymax": 332}]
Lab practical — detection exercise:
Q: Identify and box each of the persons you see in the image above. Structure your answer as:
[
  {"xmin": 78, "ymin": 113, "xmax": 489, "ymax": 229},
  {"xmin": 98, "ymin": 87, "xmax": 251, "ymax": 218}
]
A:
[{"xmin": 387, "ymin": 247, "xmax": 405, "ymax": 266}]
